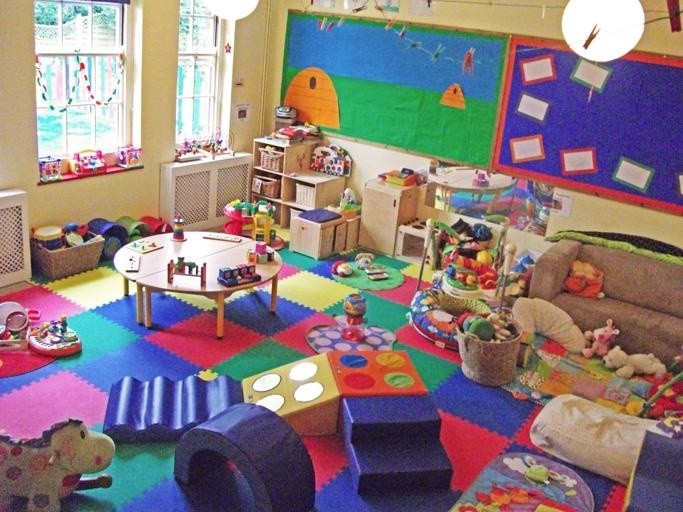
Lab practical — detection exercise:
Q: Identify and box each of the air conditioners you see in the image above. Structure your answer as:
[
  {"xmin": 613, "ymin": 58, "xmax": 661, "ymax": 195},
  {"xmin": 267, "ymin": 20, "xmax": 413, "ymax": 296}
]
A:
[
  {"xmin": 0, "ymin": 189, "xmax": 33, "ymax": 288},
  {"xmin": 161, "ymin": 152, "xmax": 254, "ymax": 232}
]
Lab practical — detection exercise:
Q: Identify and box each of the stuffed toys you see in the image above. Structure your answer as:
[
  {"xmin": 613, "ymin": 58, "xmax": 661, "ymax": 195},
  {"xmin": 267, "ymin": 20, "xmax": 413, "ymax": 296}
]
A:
[
  {"xmin": 625, "ymin": 355, "xmax": 683, "ymax": 419},
  {"xmin": 1, "ymin": 417, "xmax": 116, "ymax": 512},
  {"xmin": 510, "ymin": 297, "xmax": 590, "ymax": 354},
  {"xmin": 563, "ymin": 260, "xmax": 605, "ymax": 299},
  {"xmin": 601, "ymin": 345, "xmax": 667, "ymax": 380},
  {"xmin": 582, "ymin": 318, "xmax": 620, "ymax": 359}
]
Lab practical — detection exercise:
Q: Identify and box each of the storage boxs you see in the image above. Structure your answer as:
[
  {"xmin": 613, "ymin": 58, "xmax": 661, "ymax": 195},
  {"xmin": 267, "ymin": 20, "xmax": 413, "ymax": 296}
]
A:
[
  {"xmin": 326, "ymin": 206, "xmax": 361, "ymax": 250},
  {"xmin": 295, "ymin": 183, "xmax": 315, "ymax": 206},
  {"xmin": 289, "ymin": 209, "xmax": 303, "ymax": 230},
  {"xmin": 290, "ymin": 217, "xmax": 347, "ymax": 260}
]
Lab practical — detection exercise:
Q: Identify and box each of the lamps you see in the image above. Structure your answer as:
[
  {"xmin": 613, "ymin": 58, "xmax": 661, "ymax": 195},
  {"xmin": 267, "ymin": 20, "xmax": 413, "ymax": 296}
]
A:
[{"xmin": 562, "ymin": 0, "xmax": 683, "ymax": 64}]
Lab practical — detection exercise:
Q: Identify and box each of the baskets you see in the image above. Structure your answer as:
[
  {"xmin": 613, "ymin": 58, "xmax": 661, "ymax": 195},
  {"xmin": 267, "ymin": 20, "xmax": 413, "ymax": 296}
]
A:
[{"xmin": 455, "ymin": 307, "xmax": 523, "ymax": 387}]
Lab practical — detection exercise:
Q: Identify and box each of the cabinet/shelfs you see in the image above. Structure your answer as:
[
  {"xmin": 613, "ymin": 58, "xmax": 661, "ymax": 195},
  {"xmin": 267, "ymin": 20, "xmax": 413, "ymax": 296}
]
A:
[{"xmin": 251, "ymin": 138, "xmax": 346, "ymax": 231}]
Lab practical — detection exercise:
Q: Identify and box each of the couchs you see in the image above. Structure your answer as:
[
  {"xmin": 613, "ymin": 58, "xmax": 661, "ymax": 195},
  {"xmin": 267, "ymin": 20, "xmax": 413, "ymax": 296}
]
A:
[{"xmin": 529, "ymin": 239, "xmax": 683, "ymax": 371}]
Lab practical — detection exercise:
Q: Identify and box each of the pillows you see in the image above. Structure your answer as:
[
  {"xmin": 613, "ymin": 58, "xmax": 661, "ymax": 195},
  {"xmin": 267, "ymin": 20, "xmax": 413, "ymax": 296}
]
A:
[{"xmin": 530, "ymin": 394, "xmax": 673, "ymax": 486}]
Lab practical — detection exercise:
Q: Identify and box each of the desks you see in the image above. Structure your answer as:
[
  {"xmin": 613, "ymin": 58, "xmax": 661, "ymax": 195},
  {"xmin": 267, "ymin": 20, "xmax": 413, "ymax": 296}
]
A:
[
  {"xmin": 113, "ymin": 240, "xmax": 283, "ymax": 339},
  {"xmin": 107, "ymin": 233, "xmax": 253, "ymax": 334},
  {"xmin": 429, "ymin": 167, "xmax": 519, "ymax": 214}
]
